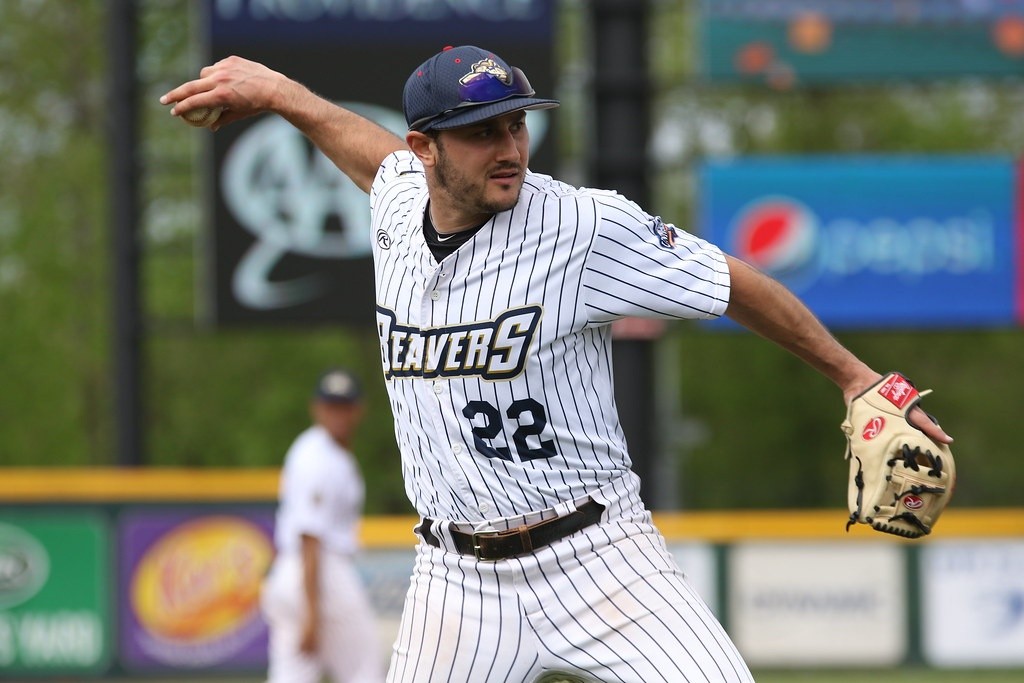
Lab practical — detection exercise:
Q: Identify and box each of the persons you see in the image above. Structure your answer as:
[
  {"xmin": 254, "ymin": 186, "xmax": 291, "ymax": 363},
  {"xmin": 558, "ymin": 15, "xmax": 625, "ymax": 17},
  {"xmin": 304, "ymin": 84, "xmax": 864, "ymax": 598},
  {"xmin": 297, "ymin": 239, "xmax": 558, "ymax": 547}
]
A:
[
  {"xmin": 265, "ymin": 373, "xmax": 383, "ymax": 683},
  {"xmin": 158, "ymin": 45, "xmax": 955, "ymax": 683}
]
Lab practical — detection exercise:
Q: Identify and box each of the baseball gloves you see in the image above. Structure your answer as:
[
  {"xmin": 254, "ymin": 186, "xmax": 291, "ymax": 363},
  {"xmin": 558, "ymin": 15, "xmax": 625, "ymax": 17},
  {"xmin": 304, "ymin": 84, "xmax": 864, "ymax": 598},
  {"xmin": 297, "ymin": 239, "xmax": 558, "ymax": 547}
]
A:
[{"xmin": 841, "ymin": 370, "xmax": 957, "ymax": 540}]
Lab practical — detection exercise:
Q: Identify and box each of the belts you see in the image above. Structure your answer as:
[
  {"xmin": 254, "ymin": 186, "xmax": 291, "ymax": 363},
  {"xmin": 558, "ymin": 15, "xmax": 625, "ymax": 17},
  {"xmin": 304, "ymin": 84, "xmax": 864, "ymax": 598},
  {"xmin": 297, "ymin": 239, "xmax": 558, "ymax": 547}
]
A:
[{"xmin": 422, "ymin": 501, "xmax": 604, "ymax": 560}]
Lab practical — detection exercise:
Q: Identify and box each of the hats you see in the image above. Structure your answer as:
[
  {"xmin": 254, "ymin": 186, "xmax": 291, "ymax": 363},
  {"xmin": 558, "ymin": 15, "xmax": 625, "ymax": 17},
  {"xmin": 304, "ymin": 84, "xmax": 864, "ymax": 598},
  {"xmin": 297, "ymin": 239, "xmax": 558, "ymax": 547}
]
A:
[
  {"xmin": 314, "ymin": 367, "xmax": 360, "ymax": 407},
  {"xmin": 400, "ymin": 44, "xmax": 560, "ymax": 134}
]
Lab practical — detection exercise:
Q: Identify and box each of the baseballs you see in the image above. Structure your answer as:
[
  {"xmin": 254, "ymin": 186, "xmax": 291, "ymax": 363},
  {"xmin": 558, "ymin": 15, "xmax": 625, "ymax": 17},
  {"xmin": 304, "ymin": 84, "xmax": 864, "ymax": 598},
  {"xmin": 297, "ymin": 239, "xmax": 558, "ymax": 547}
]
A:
[{"xmin": 172, "ymin": 89, "xmax": 224, "ymax": 129}]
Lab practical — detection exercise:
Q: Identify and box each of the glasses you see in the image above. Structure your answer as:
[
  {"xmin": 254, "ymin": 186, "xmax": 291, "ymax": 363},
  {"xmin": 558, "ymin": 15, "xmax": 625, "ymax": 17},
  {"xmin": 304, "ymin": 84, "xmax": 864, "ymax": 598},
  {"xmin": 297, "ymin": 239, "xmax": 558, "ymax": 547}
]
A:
[{"xmin": 408, "ymin": 64, "xmax": 537, "ymax": 128}]
